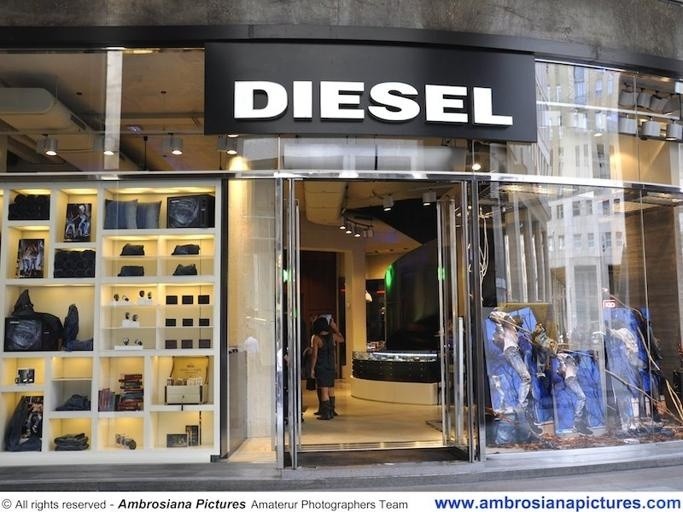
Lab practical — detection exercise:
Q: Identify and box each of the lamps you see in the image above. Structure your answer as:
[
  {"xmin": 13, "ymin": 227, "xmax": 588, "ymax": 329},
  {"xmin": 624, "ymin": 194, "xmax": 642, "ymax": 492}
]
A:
[
  {"xmin": 339, "ymin": 212, "xmax": 376, "ymax": 241},
  {"xmin": 380, "ymin": 187, "xmax": 437, "ymax": 212},
  {"xmin": 618, "ymin": 79, "xmax": 679, "ymax": 114},
  {"xmin": 537, "ymin": 105, "xmax": 682, "ymax": 142},
  {"xmin": 30, "ymin": 126, "xmax": 236, "ymax": 160},
  {"xmin": 469, "ymin": 153, "xmax": 483, "ymax": 171}
]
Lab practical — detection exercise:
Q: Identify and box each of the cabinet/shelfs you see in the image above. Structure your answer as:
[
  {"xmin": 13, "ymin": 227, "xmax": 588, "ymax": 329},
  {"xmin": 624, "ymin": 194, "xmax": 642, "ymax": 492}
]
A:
[{"xmin": 0, "ymin": 177, "xmax": 229, "ymax": 467}]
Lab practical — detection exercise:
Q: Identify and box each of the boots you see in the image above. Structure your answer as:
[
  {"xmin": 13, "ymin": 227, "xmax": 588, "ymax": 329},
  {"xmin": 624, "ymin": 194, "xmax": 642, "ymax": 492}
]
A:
[{"xmin": 314, "ymin": 396, "xmax": 335, "ymax": 419}]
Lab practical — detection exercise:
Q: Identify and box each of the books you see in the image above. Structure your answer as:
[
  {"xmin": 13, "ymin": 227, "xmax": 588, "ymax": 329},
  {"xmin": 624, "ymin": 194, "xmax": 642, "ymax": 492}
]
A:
[
  {"xmin": 167, "ymin": 433, "xmax": 190, "ymax": 447},
  {"xmin": 186, "ymin": 425, "xmax": 200, "ymax": 446},
  {"xmin": 98, "ymin": 373, "xmax": 144, "ymax": 411}
]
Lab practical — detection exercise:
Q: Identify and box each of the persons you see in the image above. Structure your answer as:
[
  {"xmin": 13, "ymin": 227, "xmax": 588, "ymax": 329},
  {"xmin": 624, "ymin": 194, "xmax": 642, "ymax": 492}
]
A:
[
  {"xmin": 606, "ymin": 318, "xmax": 648, "ymax": 421},
  {"xmin": 487, "ymin": 311, "xmax": 544, "ymax": 437},
  {"xmin": 494, "ymin": 377, "xmax": 507, "ymax": 410},
  {"xmin": 66, "ymin": 205, "xmax": 91, "ymax": 239},
  {"xmin": 557, "ymin": 353, "xmax": 594, "ymax": 437},
  {"xmin": 21, "ymin": 240, "xmax": 44, "ymax": 275},
  {"xmin": 283, "ymin": 311, "xmax": 346, "ymax": 426}
]
